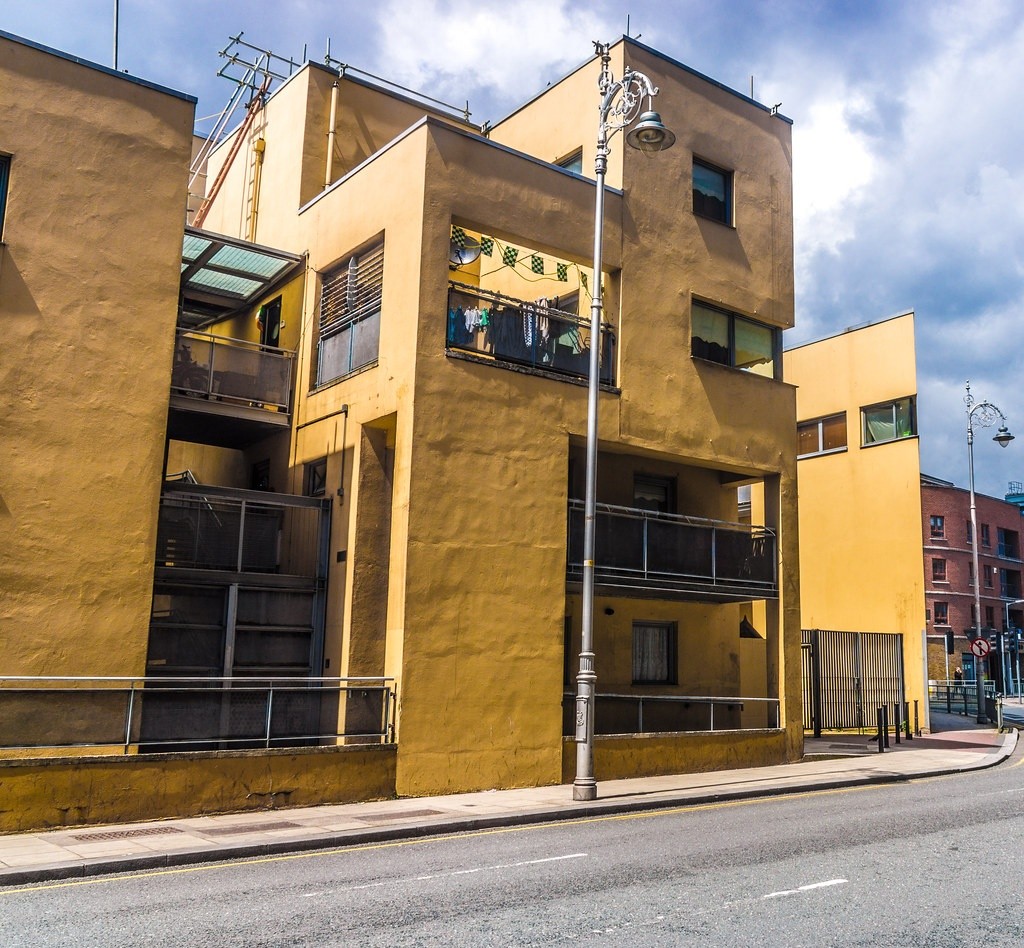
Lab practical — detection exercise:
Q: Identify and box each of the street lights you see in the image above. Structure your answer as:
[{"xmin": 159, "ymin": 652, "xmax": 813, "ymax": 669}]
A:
[
  {"xmin": 1004, "ymin": 599, "xmax": 1024, "ymax": 695},
  {"xmin": 571, "ymin": 64, "xmax": 677, "ymax": 799},
  {"xmin": 966, "ymin": 399, "xmax": 1017, "ymax": 723}
]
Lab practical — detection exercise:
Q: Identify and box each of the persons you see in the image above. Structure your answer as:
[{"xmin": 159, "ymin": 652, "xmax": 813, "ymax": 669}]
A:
[{"xmin": 954, "ymin": 667, "xmax": 962, "ymax": 693}]
